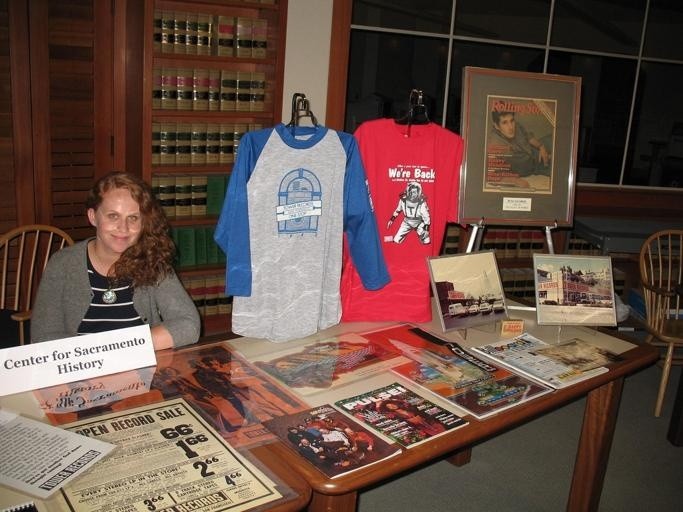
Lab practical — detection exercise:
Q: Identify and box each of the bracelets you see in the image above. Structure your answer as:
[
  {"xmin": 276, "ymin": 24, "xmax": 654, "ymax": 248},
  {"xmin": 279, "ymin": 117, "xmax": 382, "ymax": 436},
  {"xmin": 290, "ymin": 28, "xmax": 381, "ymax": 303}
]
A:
[{"xmin": 536, "ymin": 143, "xmax": 544, "ymax": 152}]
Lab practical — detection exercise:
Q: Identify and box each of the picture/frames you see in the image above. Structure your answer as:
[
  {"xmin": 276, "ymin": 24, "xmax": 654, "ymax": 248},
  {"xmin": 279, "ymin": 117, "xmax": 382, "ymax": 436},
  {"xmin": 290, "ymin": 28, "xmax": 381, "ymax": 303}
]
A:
[
  {"xmin": 454, "ymin": 63, "xmax": 584, "ymax": 229},
  {"xmin": 426, "ymin": 248, "xmax": 509, "ymax": 335},
  {"xmin": 531, "ymin": 251, "xmax": 618, "ymax": 327}
]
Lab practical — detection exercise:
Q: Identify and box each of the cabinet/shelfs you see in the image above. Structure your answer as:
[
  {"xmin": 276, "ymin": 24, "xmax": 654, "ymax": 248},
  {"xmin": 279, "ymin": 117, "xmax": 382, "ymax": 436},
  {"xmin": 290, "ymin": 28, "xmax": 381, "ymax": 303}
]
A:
[
  {"xmin": 126, "ymin": 0, "xmax": 289, "ymax": 342},
  {"xmin": 0, "ymin": 0, "xmax": 125, "ymax": 312}
]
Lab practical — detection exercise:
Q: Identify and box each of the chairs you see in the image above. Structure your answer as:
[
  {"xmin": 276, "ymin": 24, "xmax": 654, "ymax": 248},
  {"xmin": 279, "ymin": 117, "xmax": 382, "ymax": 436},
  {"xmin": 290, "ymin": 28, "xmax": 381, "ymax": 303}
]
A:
[
  {"xmin": 0, "ymin": 223, "xmax": 75, "ymax": 346},
  {"xmin": 638, "ymin": 224, "xmax": 681, "ymax": 420}
]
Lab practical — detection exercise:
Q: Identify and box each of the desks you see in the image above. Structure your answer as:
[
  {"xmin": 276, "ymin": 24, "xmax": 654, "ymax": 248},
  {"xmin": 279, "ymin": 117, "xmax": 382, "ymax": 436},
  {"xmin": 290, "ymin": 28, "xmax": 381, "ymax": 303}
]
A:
[{"xmin": 1, "ymin": 292, "xmax": 661, "ymax": 512}]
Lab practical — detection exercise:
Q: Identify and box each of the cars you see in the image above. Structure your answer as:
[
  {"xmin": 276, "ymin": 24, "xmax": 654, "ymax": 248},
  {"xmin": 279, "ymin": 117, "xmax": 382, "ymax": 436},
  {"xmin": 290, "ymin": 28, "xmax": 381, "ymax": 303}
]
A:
[{"xmin": 467, "ymin": 300, "xmax": 504, "ymax": 315}]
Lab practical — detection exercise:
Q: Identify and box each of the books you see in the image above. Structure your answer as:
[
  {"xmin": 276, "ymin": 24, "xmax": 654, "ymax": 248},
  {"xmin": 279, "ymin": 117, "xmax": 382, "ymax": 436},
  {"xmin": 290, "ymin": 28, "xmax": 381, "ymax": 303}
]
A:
[
  {"xmin": 472, "ymin": 331, "xmax": 608, "ymax": 388},
  {"xmin": 333, "ymin": 382, "xmax": 469, "ymax": 448},
  {"xmin": 260, "ymin": 403, "xmax": 403, "ymax": 480},
  {"xmin": 389, "ymin": 346, "xmax": 553, "ymax": 419}
]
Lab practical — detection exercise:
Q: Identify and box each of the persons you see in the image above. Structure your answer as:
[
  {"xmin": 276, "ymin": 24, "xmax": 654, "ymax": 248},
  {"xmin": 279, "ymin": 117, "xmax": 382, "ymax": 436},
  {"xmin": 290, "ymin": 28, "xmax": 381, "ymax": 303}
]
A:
[
  {"xmin": 489, "ymin": 105, "xmax": 551, "ymax": 190},
  {"xmin": 142, "ymin": 353, "xmax": 295, "ymax": 437},
  {"xmin": 31, "ymin": 170, "xmax": 201, "ymax": 350},
  {"xmin": 377, "ymin": 397, "xmax": 446, "ymax": 436},
  {"xmin": 286, "ymin": 414, "xmax": 377, "ymax": 473}
]
